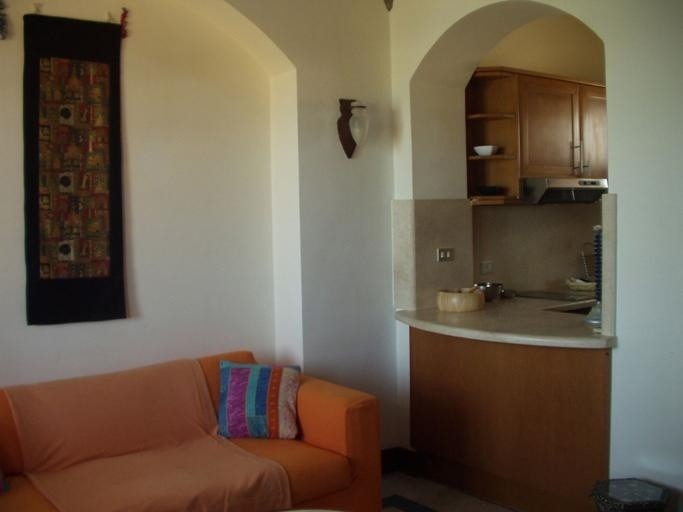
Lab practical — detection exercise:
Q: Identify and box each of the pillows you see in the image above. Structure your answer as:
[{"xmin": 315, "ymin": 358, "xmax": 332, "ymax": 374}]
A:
[{"xmin": 215, "ymin": 358, "xmax": 302, "ymax": 441}]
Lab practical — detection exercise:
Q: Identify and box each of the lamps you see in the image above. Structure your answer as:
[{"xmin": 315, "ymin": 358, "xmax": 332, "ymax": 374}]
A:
[{"xmin": 336, "ymin": 98, "xmax": 370, "ymax": 161}]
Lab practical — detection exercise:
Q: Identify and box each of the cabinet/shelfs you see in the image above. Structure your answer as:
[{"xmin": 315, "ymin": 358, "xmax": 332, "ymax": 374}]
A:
[
  {"xmin": 464, "ymin": 65, "xmax": 525, "ymax": 209},
  {"xmin": 521, "ymin": 70, "xmax": 608, "ymax": 180}
]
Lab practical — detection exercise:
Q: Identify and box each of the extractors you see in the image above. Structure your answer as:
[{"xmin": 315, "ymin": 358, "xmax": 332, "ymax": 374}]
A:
[{"xmin": 523, "ymin": 177, "xmax": 610, "ymax": 205}]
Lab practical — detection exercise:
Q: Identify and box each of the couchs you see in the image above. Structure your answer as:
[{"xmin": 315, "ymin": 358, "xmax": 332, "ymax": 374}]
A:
[{"xmin": 1, "ymin": 350, "xmax": 384, "ymax": 512}]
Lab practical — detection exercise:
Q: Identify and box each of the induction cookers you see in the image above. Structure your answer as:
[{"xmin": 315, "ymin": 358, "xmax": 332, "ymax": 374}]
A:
[{"xmin": 517, "ymin": 290, "xmax": 596, "ymax": 302}]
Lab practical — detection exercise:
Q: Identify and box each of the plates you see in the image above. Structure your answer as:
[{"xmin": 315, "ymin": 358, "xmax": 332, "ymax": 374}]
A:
[{"xmin": 566, "ymin": 277, "xmax": 597, "ymax": 291}]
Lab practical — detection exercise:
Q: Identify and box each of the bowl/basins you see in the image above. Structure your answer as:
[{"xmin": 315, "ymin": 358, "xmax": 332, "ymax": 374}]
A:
[
  {"xmin": 473, "ymin": 283, "xmax": 503, "ymax": 302},
  {"xmin": 436, "ymin": 288, "xmax": 484, "ymax": 313},
  {"xmin": 472, "ymin": 144, "xmax": 498, "ymax": 156}
]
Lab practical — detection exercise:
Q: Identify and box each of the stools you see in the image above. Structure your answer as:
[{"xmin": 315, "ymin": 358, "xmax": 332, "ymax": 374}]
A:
[{"xmin": 588, "ymin": 477, "xmax": 671, "ymax": 511}]
